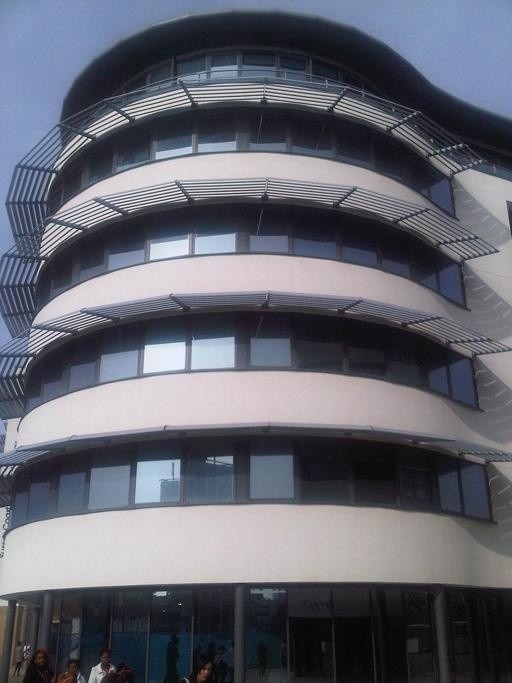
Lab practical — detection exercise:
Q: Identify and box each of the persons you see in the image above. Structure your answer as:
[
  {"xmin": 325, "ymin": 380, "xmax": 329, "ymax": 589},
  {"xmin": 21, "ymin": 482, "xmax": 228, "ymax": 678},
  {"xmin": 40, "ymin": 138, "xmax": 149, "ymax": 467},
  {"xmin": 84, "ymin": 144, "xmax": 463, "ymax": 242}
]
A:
[
  {"xmin": 54, "ymin": 673, "xmax": 79, "ymax": 682},
  {"xmin": 88, "ymin": 650, "xmax": 115, "ymax": 682},
  {"xmin": 13, "ymin": 641, "xmax": 23, "ymax": 675},
  {"xmin": 23, "ymin": 648, "xmax": 53, "ymax": 682},
  {"xmin": 100, "ymin": 663, "xmax": 135, "ymax": 682},
  {"xmin": 164, "ymin": 632, "xmax": 268, "ymax": 682},
  {"xmin": 56, "ymin": 658, "xmax": 85, "ymax": 682}
]
